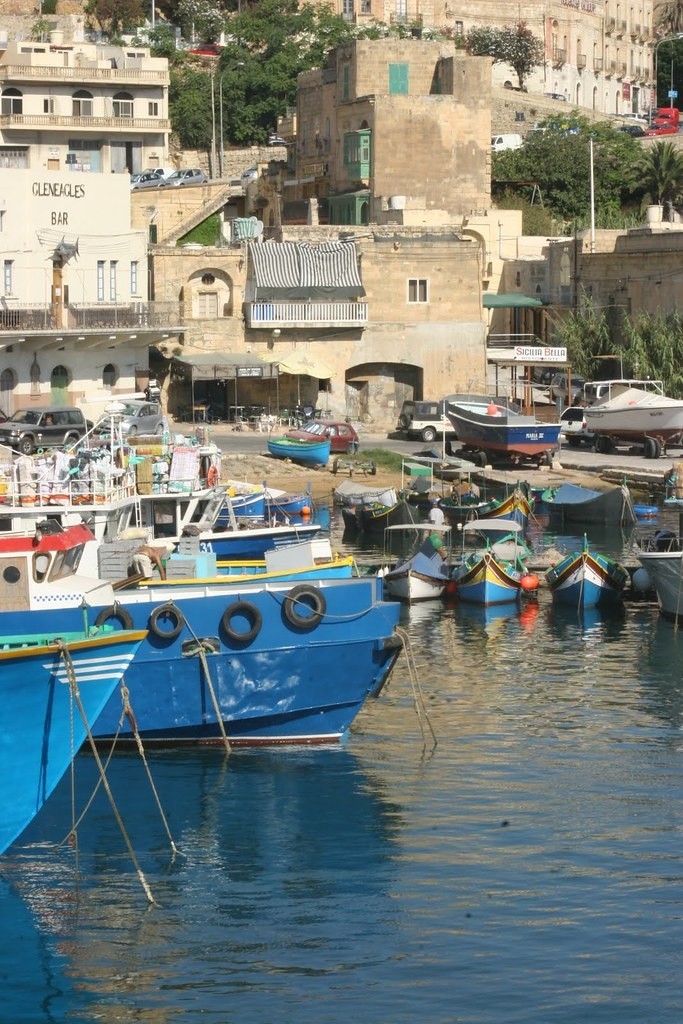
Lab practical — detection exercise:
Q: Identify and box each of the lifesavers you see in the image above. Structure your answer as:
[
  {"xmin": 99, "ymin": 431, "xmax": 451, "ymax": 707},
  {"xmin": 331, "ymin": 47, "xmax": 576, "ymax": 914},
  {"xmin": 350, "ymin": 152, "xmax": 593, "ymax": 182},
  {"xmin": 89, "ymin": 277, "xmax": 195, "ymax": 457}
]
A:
[
  {"xmin": 222, "ymin": 601, "xmax": 262, "ymax": 639},
  {"xmin": 285, "ymin": 584, "xmax": 326, "ymax": 629},
  {"xmin": 150, "ymin": 605, "xmax": 184, "ymax": 638},
  {"xmin": 207, "ymin": 465, "xmax": 218, "ymax": 487},
  {"xmin": 94, "ymin": 608, "xmax": 133, "ymax": 632}
]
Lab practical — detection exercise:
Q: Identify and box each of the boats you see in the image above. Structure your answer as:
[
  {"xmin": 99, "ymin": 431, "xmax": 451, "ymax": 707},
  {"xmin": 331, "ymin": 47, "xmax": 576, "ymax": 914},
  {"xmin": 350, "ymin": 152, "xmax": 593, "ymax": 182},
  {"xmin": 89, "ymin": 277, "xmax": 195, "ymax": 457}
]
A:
[
  {"xmin": 0, "ymin": 400, "xmax": 409, "ymax": 856},
  {"xmin": 267, "ymin": 437, "xmax": 331, "ymax": 468},
  {"xmin": 445, "ymin": 395, "xmax": 562, "ymax": 464},
  {"xmin": 342, "ymin": 463, "xmax": 683, "ymax": 629},
  {"xmin": 583, "ymin": 384, "xmax": 683, "ymax": 446}
]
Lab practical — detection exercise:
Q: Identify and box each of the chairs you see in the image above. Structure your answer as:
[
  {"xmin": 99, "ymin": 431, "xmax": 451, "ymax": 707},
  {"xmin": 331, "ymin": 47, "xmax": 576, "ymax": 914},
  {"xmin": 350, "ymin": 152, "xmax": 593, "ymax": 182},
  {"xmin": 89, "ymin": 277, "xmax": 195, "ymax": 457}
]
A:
[
  {"xmin": 234, "ymin": 414, "xmax": 278, "ymax": 433},
  {"xmin": 280, "ymin": 405, "xmax": 322, "ymax": 426}
]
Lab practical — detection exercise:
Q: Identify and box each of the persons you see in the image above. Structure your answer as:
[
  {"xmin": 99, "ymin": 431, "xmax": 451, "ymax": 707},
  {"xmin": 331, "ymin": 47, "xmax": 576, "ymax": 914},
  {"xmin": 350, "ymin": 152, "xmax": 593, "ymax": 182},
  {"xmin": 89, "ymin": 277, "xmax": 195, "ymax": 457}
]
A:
[
  {"xmin": 428, "ymin": 499, "xmax": 445, "ymax": 544},
  {"xmin": 43, "ymin": 414, "xmax": 54, "ymax": 425},
  {"xmin": 133, "ymin": 545, "xmax": 166, "ymax": 581}
]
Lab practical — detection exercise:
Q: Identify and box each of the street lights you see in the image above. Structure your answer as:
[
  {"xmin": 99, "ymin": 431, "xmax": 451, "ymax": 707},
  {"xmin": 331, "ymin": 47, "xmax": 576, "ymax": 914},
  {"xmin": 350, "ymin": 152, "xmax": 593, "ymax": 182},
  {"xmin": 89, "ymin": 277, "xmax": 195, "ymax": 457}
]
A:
[
  {"xmin": 654, "ymin": 33, "xmax": 683, "ymax": 108},
  {"xmin": 218, "ymin": 62, "xmax": 244, "ymax": 178},
  {"xmin": 590, "ymin": 133, "xmax": 597, "ymax": 253},
  {"xmin": 543, "ymin": 10, "xmax": 552, "ymax": 93},
  {"xmin": 209, "ymin": 73, "xmax": 217, "ymax": 179}
]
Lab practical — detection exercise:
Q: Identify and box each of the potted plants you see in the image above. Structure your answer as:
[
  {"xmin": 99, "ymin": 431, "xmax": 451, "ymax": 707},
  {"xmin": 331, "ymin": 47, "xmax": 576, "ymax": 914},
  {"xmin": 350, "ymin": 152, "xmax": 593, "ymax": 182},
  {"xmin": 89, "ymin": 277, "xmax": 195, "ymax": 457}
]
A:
[
  {"xmin": 410, "ymin": 18, "xmax": 422, "ymax": 40},
  {"xmin": 388, "ymin": 24, "xmax": 399, "ymax": 39},
  {"xmin": 423, "ymin": 26, "xmax": 448, "ymax": 41}
]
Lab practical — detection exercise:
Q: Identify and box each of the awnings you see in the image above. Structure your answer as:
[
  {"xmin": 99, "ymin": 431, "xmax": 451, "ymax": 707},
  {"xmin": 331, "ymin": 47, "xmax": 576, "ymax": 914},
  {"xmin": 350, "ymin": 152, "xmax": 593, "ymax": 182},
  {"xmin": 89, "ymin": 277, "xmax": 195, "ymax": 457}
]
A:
[
  {"xmin": 249, "ymin": 240, "xmax": 363, "ymax": 288},
  {"xmin": 483, "ymin": 293, "xmax": 543, "ymax": 308},
  {"xmin": 171, "ymin": 351, "xmax": 279, "ymax": 379},
  {"xmin": 275, "ymin": 350, "xmax": 334, "ymax": 379}
]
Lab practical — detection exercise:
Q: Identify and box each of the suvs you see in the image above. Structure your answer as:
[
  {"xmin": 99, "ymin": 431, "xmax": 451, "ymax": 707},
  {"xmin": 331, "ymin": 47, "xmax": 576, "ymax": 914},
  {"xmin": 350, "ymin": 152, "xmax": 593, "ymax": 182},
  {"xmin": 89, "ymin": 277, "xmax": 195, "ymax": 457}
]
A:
[
  {"xmin": 396, "ymin": 400, "xmax": 457, "ymax": 442},
  {"xmin": 559, "ymin": 407, "xmax": 613, "ymax": 448}
]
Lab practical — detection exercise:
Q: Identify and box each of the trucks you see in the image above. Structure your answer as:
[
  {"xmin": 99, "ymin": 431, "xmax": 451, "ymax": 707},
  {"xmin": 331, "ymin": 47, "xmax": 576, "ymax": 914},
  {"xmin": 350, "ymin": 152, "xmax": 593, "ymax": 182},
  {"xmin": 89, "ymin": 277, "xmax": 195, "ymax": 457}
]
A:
[{"xmin": 525, "ymin": 119, "xmax": 579, "ymax": 145}]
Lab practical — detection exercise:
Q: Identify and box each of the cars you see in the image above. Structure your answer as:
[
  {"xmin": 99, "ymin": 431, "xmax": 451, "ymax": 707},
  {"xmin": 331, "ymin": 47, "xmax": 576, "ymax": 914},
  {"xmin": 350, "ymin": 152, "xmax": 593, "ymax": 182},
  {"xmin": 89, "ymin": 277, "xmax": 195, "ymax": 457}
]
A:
[
  {"xmin": 130, "ymin": 172, "xmax": 166, "ymax": 189},
  {"xmin": 624, "ymin": 113, "xmax": 648, "ymax": 124},
  {"xmin": 158, "ymin": 169, "xmax": 208, "ymax": 187},
  {"xmin": 143, "ymin": 168, "xmax": 175, "ymax": 180},
  {"xmin": 608, "ymin": 126, "xmax": 645, "ymax": 138},
  {"xmin": 241, "ymin": 165, "xmax": 266, "ymax": 179},
  {"xmin": 644, "ymin": 123, "xmax": 678, "ymax": 136},
  {"xmin": 185, "ymin": 44, "xmax": 224, "ymax": 55},
  {"xmin": 286, "ymin": 420, "xmax": 360, "ymax": 455}
]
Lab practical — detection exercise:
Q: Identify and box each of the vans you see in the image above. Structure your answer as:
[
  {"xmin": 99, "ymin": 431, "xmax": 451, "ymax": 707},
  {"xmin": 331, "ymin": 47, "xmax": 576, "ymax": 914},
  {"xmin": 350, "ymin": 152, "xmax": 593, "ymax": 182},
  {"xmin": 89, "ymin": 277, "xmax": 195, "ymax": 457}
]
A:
[
  {"xmin": 652, "ymin": 108, "xmax": 679, "ymax": 127},
  {"xmin": 0, "ymin": 407, "xmax": 87, "ymax": 455},
  {"xmin": 95, "ymin": 400, "xmax": 164, "ymax": 436},
  {"xmin": 551, "ymin": 374, "xmax": 587, "ymax": 407},
  {"xmin": 491, "ymin": 134, "xmax": 522, "ymax": 152}
]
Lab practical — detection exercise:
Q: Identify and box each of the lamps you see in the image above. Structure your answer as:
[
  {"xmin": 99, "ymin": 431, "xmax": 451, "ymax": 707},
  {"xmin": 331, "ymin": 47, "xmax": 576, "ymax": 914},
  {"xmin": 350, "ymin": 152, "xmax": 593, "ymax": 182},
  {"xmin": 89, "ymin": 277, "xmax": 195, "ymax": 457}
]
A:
[{"xmin": 271, "ymin": 329, "xmax": 281, "ymax": 347}]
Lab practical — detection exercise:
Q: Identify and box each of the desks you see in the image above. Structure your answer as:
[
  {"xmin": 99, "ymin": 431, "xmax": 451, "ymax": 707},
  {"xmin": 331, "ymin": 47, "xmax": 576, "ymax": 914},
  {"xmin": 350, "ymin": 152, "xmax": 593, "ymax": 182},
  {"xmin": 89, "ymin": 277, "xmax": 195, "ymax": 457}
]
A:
[
  {"xmin": 227, "ymin": 406, "xmax": 245, "ymax": 420},
  {"xmin": 189, "ymin": 406, "xmax": 206, "ymax": 421}
]
